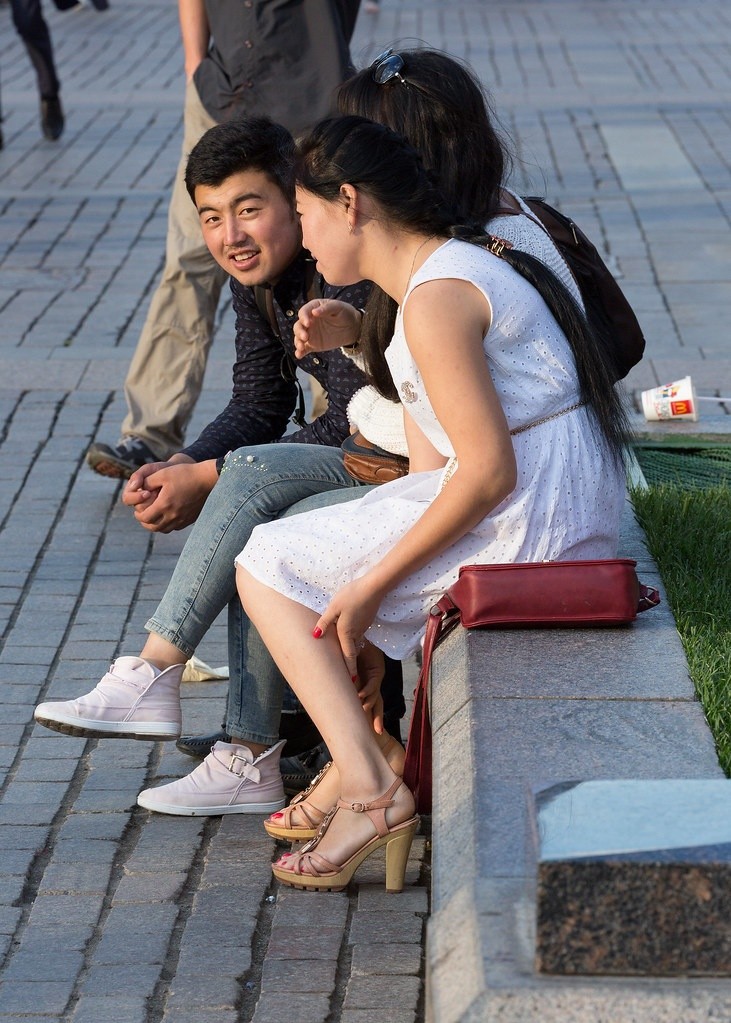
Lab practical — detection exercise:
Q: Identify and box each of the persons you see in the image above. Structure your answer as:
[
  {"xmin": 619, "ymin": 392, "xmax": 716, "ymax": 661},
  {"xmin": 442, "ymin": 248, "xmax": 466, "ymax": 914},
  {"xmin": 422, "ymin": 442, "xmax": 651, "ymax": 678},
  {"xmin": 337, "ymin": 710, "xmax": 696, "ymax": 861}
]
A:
[
  {"xmin": 235, "ymin": 114, "xmax": 622, "ymax": 894},
  {"xmin": 0, "ymin": 0, "xmax": 583, "ymax": 815}
]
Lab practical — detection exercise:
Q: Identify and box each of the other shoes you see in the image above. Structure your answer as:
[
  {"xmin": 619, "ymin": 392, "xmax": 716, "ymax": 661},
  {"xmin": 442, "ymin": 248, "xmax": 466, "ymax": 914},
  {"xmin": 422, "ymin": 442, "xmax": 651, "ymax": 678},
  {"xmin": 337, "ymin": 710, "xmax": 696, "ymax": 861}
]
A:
[
  {"xmin": 279, "ymin": 742, "xmax": 333, "ymax": 795},
  {"xmin": 41, "ymin": 93, "xmax": 64, "ymax": 141},
  {"xmin": 87, "ymin": 435, "xmax": 162, "ymax": 479},
  {"xmin": 176, "ymin": 725, "xmax": 231, "ymax": 759}
]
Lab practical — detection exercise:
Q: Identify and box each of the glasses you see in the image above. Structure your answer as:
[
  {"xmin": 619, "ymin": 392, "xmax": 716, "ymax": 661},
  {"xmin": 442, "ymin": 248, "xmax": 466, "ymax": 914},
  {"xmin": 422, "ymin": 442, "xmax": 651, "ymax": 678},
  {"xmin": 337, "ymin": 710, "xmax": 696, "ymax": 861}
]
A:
[{"xmin": 369, "ymin": 48, "xmax": 411, "ymax": 92}]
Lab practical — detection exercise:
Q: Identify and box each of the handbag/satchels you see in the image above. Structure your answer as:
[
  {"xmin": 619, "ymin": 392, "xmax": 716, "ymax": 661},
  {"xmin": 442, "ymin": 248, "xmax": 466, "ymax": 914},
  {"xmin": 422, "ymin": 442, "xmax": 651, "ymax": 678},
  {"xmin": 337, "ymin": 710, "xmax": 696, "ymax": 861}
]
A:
[
  {"xmin": 341, "ymin": 431, "xmax": 410, "ymax": 485},
  {"xmin": 406, "ymin": 558, "xmax": 660, "ymax": 800},
  {"xmin": 521, "ymin": 195, "xmax": 646, "ymax": 384}
]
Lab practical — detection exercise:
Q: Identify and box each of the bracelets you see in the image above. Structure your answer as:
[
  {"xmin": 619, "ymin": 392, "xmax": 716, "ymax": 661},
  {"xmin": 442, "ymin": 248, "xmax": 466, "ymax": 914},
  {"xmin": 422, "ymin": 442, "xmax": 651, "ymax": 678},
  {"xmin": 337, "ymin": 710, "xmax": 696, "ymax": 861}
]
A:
[{"xmin": 344, "ymin": 309, "xmax": 364, "ymax": 350}]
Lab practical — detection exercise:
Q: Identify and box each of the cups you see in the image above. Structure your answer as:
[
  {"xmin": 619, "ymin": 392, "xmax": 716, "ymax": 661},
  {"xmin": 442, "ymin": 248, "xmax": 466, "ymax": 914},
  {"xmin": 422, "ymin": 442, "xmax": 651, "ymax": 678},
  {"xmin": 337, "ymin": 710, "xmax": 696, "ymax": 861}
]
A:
[{"xmin": 641, "ymin": 376, "xmax": 700, "ymax": 422}]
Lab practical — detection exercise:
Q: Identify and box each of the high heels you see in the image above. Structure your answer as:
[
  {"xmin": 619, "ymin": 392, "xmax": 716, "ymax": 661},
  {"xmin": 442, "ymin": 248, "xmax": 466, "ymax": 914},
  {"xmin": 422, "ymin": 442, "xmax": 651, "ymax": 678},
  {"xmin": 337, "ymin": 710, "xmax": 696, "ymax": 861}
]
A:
[
  {"xmin": 264, "ymin": 736, "xmax": 408, "ymax": 844},
  {"xmin": 271, "ymin": 777, "xmax": 419, "ymax": 893}
]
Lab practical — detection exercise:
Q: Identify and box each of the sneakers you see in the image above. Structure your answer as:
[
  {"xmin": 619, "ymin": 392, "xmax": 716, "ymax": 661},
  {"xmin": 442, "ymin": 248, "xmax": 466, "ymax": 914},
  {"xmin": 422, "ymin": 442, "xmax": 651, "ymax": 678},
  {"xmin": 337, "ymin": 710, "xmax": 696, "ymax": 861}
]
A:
[
  {"xmin": 137, "ymin": 739, "xmax": 286, "ymax": 816},
  {"xmin": 34, "ymin": 655, "xmax": 186, "ymax": 741}
]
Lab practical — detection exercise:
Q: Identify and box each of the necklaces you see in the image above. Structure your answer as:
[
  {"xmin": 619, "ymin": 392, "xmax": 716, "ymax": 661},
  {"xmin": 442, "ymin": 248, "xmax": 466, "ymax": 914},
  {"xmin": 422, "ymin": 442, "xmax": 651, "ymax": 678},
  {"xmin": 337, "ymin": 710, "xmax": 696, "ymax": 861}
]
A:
[{"xmin": 403, "ymin": 237, "xmax": 432, "ymax": 296}]
